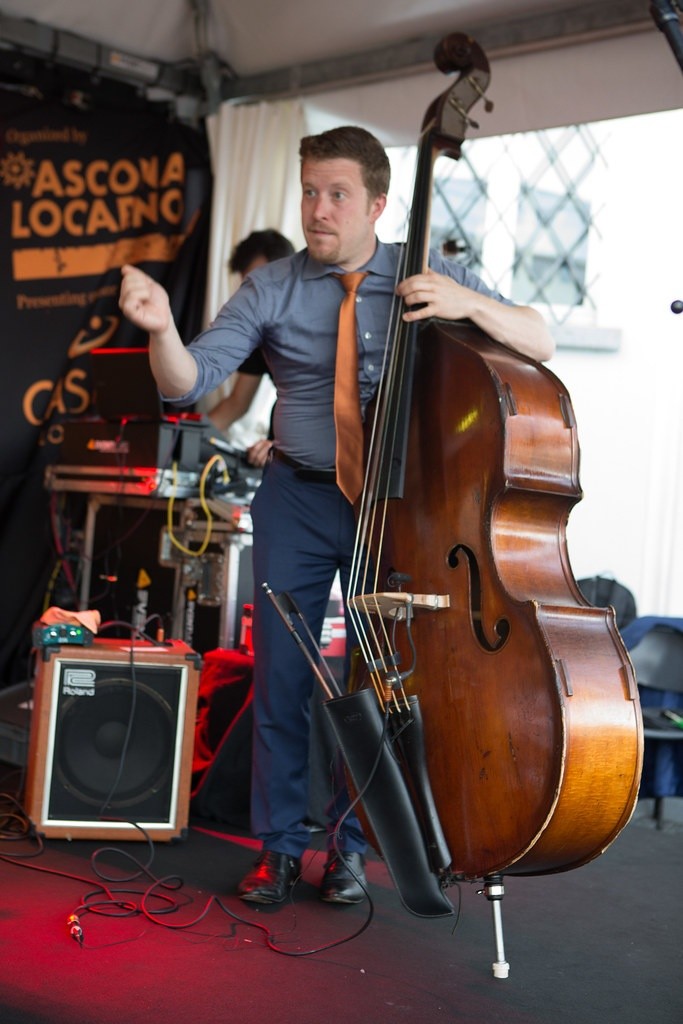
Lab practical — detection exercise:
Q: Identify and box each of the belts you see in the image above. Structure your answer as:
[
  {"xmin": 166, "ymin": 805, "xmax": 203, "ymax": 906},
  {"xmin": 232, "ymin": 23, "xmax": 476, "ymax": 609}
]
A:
[{"xmin": 271, "ymin": 446, "xmax": 335, "ymax": 484}]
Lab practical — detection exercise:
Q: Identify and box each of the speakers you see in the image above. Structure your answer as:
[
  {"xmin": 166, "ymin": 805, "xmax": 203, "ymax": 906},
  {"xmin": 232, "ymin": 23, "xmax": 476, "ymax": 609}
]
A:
[{"xmin": 24, "ymin": 638, "xmax": 201, "ymax": 843}]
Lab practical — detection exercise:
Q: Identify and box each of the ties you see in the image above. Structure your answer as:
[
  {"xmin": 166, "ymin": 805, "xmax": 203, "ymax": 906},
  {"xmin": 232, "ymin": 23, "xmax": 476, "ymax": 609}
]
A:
[{"xmin": 327, "ymin": 271, "xmax": 366, "ymax": 504}]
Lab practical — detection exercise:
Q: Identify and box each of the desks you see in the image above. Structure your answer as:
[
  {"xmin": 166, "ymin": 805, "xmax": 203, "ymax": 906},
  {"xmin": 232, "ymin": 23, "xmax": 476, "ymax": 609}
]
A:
[{"xmin": 80, "ymin": 492, "xmax": 236, "ymax": 646}]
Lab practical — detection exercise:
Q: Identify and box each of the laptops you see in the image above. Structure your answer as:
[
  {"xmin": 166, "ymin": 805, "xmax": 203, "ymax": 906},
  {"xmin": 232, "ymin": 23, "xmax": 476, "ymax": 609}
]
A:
[{"xmin": 89, "ymin": 349, "xmax": 209, "ymax": 427}]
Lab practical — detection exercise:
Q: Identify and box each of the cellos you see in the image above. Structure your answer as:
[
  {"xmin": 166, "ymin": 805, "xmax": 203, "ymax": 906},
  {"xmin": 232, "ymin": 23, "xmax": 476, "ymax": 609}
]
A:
[{"xmin": 335, "ymin": 29, "xmax": 645, "ymax": 979}]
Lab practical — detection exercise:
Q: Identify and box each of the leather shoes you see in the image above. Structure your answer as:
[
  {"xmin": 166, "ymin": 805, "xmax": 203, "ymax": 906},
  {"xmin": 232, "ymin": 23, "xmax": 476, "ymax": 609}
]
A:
[
  {"xmin": 317, "ymin": 849, "xmax": 366, "ymax": 904},
  {"xmin": 240, "ymin": 849, "xmax": 302, "ymax": 904}
]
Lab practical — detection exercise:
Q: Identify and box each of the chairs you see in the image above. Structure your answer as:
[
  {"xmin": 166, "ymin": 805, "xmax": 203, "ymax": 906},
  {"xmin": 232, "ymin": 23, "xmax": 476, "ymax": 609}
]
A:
[{"xmin": 577, "ymin": 577, "xmax": 683, "ymax": 830}]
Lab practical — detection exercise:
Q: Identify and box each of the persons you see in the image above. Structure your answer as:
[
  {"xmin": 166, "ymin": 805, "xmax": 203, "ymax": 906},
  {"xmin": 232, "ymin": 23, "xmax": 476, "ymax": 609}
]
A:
[
  {"xmin": 207, "ymin": 228, "xmax": 297, "ymax": 465},
  {"xmin": 119, "ymin": 126, "xmax": 555, "ymax": 905}
]
